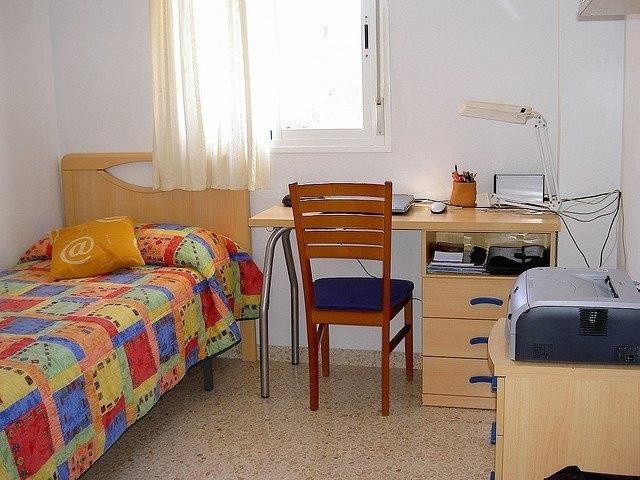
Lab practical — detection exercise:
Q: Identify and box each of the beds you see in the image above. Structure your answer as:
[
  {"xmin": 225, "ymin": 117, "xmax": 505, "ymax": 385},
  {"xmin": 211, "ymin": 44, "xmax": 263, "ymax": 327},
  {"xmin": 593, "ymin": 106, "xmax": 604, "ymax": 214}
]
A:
[{"xmin": 0, "ymin": 152, "xmax": 265, "ymax": 480}]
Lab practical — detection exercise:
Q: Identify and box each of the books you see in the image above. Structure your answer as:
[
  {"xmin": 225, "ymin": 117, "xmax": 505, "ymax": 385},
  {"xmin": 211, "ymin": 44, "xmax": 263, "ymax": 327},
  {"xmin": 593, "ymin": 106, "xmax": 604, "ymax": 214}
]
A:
[{"xmin": 428, "ymin": 242, "xmax": 486, "ymax": 275}]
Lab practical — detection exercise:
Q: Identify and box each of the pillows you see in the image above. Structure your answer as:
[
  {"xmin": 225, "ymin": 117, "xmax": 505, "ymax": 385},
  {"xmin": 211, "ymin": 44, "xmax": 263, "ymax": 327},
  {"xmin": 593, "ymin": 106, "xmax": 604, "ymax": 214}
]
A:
[{"xmin": 48, "ymin": 213, "xmax": 145, "ymax": 281}]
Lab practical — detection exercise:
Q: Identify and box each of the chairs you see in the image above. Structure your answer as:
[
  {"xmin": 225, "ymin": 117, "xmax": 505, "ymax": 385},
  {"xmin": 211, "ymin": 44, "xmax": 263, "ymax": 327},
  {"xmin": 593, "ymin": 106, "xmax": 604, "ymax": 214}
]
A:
[{"xmin": 289, "ymin": 180, "xmax": 414, "ymax": 416}]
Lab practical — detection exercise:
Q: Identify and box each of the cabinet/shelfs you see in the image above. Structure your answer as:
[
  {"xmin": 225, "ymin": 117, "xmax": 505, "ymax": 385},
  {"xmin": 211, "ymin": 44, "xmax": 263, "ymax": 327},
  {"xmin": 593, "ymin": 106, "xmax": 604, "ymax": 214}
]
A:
[{"xmin": 486, "ymin": 317, "xmax": 640, "ymax": 480}]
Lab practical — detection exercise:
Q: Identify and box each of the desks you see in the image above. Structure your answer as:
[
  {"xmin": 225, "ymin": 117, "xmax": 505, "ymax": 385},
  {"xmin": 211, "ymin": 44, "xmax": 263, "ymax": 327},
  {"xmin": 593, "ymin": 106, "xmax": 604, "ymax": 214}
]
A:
[{"xmin": 248, "ymin": 199, "xmax": 562, "ymax": 411}]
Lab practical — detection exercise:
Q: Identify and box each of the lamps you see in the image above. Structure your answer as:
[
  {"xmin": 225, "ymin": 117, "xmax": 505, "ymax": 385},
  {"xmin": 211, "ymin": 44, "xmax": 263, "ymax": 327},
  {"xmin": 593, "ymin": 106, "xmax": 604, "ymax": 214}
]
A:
[{"xmin": 457, "ymin": 99, "xmax": 564, "ymax": 215}]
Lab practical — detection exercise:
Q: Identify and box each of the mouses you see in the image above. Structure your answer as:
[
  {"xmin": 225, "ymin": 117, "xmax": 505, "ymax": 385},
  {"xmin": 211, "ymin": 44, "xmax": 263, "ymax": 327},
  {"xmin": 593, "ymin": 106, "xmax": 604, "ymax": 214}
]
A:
[{"xmin": 430, "ymin": 202, "xmax": 448, "ymax": 215}]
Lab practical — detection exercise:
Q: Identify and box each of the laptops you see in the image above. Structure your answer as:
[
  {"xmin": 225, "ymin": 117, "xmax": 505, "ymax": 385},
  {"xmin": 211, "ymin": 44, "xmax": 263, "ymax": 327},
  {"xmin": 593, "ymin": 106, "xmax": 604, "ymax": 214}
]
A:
[{"xmin": 334, "ymin": 193, "xmax": 416, "ymax": 214}]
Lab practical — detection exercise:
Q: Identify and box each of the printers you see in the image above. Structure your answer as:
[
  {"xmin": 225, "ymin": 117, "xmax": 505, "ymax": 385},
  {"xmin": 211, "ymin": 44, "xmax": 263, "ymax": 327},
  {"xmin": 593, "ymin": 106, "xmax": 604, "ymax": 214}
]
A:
[{"xmin": 507, "ymin": 267, "xmax": 640, "ymax": 365}]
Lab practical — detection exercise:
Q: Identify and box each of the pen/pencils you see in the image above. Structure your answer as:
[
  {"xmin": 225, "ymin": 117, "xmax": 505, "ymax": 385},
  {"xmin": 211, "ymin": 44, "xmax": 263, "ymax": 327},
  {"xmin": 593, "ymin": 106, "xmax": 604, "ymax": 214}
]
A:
[{"xmin": 451, "ymin": 165, "xmax": 477, "ymax": 182}]
